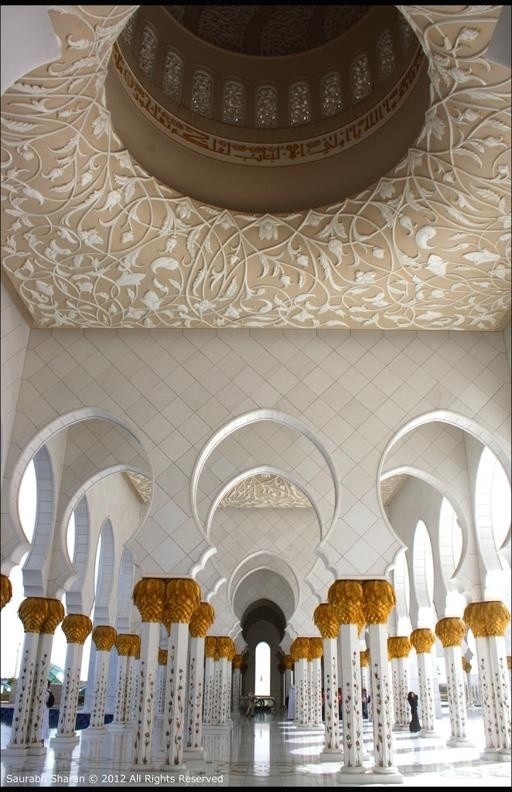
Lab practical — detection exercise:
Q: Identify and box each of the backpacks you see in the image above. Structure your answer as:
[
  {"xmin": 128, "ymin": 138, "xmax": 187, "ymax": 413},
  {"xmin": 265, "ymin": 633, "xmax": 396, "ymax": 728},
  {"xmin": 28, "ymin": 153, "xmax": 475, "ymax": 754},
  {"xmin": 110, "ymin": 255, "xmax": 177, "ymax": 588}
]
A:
[{"xmin": 46, "ymin": 690, "xmax": 54, "ymax": 707}]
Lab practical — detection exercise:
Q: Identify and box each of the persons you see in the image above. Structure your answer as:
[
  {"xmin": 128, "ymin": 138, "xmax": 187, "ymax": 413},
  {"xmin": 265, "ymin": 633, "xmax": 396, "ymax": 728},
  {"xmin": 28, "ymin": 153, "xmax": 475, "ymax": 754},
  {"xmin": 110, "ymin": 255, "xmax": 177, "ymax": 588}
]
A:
[
  {"xmin": 40, "ymin": 679, "xmax": 53, "ymax": 747},
  {"xmin": 408, "ymin": 692, "xmax": 421, "ymax": 733},
  {"xmin": 362, "ymin": 689, "xmax": 369, "ymax": 719},
  {"xmin": 320, "ymin": 687, "xmax": 342, "ymax": 722},
  {"xmin": 246, "ymin": 691, "xmax": 255, "ymax": 718}
]
